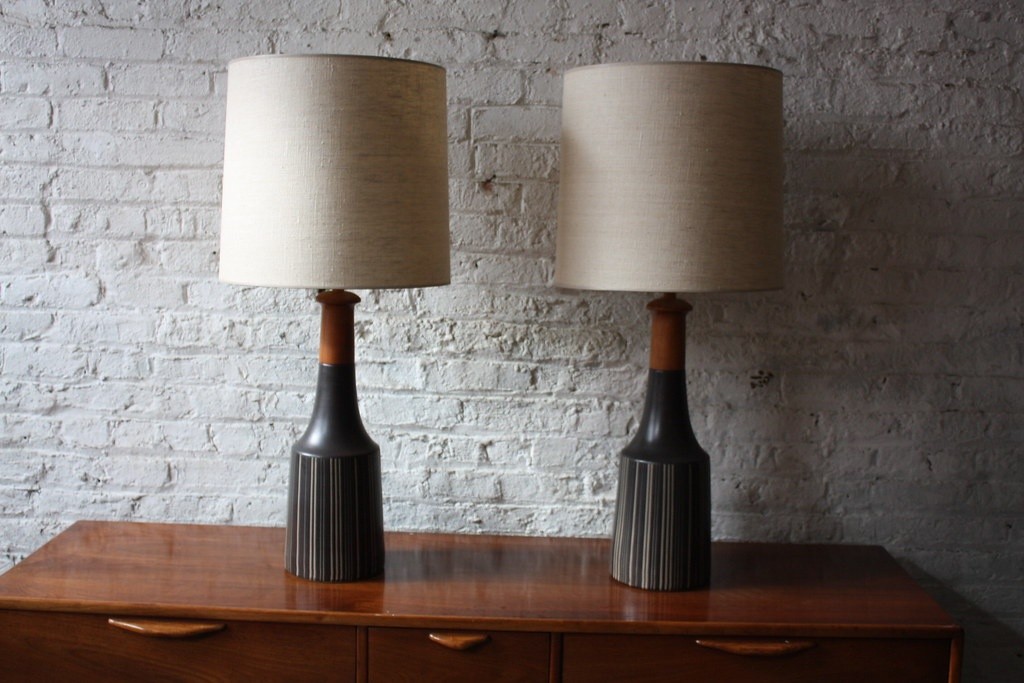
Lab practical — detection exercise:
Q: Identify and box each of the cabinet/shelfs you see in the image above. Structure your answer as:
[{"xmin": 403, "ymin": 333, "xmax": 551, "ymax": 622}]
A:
[{"xmin": 0, "ymin": 519, "xmax": 964, "ymax": 683}]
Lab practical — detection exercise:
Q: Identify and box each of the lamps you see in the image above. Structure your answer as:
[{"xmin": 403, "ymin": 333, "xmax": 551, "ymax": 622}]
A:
[
  {"xmin": 220, "ymin": 55, "xmax": 451, "ymax": 582},
  {"xmin": 553, "ymin": 61, "xmax": 784, "ymax": 594}
]
